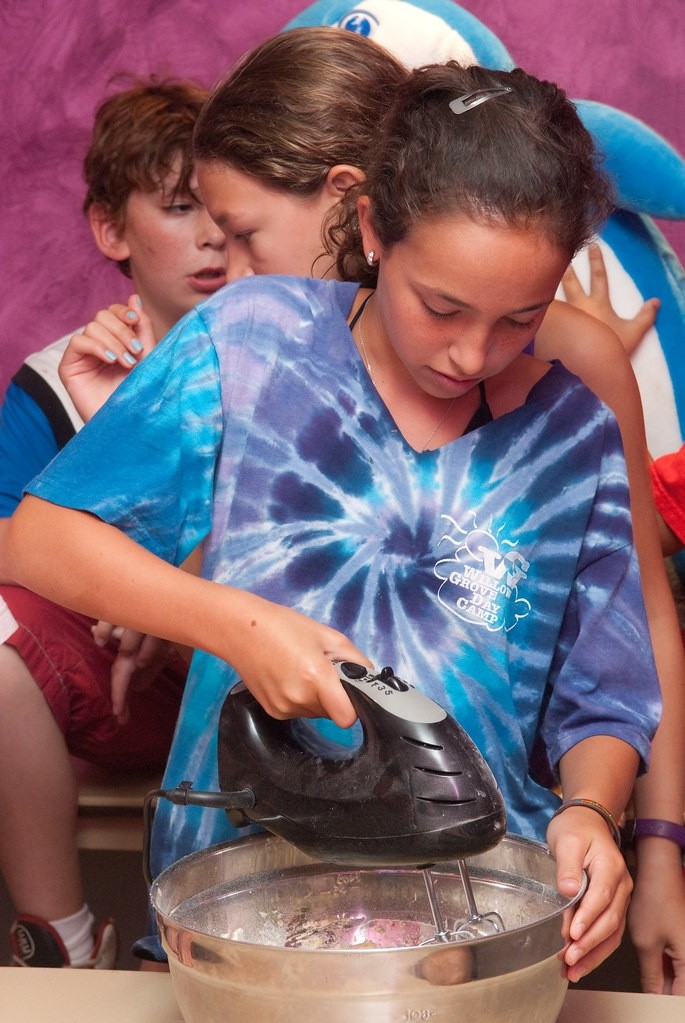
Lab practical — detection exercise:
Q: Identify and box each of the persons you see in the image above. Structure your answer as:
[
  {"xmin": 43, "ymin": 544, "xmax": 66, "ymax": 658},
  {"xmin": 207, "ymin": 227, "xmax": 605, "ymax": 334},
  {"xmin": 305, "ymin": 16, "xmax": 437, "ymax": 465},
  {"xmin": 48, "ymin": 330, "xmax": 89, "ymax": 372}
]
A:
[
  {"xmin": 6, "ymin": 65, "xmax": 663, "ymax": 981},
  {"xmin": 0, "ymin": 27, "xmax": 685, "ymax": 995}
]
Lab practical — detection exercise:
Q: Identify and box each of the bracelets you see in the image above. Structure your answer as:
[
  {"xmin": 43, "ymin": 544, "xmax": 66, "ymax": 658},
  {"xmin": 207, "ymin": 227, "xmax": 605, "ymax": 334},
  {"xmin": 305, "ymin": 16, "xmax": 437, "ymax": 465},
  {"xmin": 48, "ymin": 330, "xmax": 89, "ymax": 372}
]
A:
[
  {"xmin": 623, "ymin": 819, "xmax": 685, "ymax": 845},
  {"xmin": 552, "ymin": 799, "xmax": 621, "ymax": 847}
]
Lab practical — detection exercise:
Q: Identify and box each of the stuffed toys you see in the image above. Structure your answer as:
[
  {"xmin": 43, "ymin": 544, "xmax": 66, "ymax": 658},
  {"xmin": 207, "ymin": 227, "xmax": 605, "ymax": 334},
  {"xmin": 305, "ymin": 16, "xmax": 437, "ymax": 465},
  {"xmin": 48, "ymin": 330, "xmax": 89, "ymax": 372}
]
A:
[{"xmin": 282, "ymin": 0, "xmax": 685, "ymax": 459}]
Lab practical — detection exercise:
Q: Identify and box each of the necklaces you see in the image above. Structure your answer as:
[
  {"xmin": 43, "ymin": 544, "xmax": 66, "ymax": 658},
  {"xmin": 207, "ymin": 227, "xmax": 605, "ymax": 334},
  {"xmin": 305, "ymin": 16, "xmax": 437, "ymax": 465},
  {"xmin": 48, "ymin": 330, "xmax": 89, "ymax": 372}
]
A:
[{"xmin": 359, "ymin": 297, "xmax": 457, "ymax": 452}]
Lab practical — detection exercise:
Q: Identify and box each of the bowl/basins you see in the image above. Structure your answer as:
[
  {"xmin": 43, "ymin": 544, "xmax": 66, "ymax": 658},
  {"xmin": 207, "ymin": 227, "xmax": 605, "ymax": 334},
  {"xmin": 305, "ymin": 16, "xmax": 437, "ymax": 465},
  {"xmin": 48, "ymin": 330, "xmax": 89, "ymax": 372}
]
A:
[{"xmin": 148, "ymin": 829, "xmax": 589, "ymax": 1023}]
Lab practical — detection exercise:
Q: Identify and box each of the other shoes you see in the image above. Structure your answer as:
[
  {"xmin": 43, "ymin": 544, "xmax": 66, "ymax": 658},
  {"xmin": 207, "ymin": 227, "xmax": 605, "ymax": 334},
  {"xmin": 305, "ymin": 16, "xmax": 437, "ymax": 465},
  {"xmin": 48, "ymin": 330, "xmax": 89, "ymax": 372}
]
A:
[{"xmin": 8, "ymin": 914, "xmax": 119, "ymax": 969}]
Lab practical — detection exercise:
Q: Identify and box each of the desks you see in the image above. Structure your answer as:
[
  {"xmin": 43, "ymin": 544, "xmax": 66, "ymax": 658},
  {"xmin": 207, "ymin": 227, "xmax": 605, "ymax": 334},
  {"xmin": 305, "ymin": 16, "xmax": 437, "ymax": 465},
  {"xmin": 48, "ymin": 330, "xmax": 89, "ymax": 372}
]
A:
[{"xmin": 0, "ymin": 966, "xmax": 685, "ymax": 1023}]
